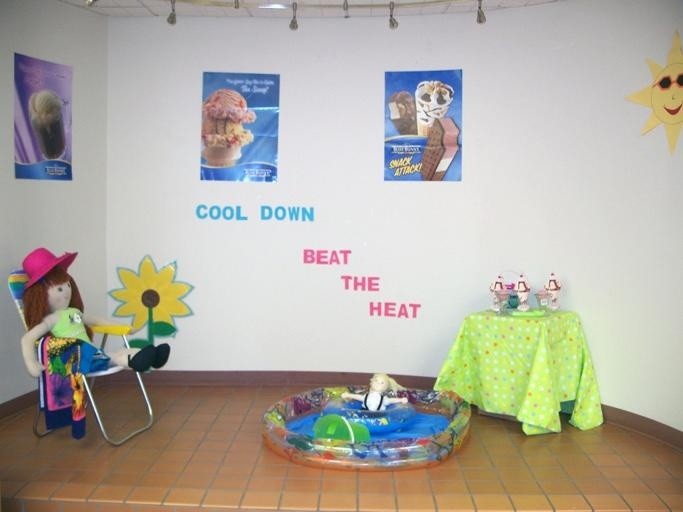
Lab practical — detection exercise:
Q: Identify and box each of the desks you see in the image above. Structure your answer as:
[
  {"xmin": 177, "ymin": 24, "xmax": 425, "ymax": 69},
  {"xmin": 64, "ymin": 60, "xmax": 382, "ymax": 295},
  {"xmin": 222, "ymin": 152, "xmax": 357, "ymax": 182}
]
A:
[{"xmin": 463, "ymin": 310, "xmax": 584, "ymax": 426}]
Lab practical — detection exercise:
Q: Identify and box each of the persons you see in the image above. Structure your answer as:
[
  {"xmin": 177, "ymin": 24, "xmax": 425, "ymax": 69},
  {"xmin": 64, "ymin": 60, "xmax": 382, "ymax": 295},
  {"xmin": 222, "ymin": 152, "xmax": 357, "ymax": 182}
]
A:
[
  {"xmin": 21, "ymin": 248, "xmax": 170, "ymax": 376},
  {"xmin": 340, "ymin": 372, "xmax": 409, "ymax": 409}
]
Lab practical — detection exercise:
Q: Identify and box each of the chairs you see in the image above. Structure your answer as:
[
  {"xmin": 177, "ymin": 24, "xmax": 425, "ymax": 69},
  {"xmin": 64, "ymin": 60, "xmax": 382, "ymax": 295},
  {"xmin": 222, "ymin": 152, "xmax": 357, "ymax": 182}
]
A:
[{"xmin": 6, "ymin": 269, "xmax": 156, "ymax": 446}]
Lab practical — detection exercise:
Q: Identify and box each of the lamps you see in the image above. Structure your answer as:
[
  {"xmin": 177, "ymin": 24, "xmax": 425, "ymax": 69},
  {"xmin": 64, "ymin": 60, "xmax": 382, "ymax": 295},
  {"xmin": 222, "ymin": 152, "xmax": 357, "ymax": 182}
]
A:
[
  {"xmin": 477, "ymin": 1, "xmax": 486, "ymax": 25},
  {"xmin": 388, "ymin": 3, "xmax": 398, "ymax": 30},
  {"xmin": 168, "ymin": 1, "xmax": 177, "ymax": 25},
  {"xmin": 290, "ymin": 3, "xmax": 297, "ymax": 30}
]
